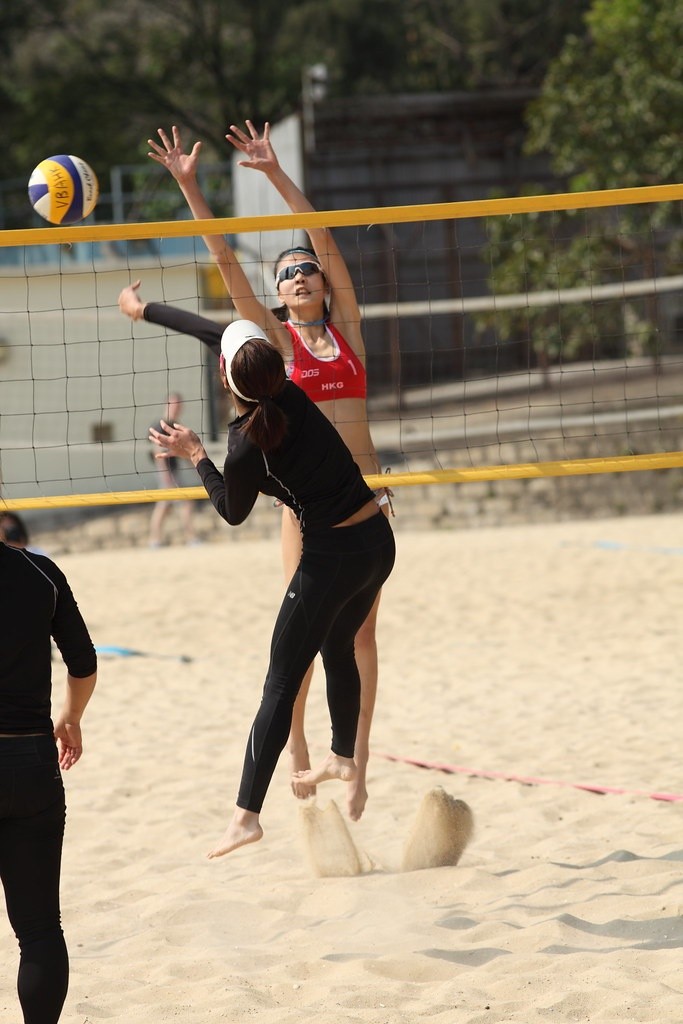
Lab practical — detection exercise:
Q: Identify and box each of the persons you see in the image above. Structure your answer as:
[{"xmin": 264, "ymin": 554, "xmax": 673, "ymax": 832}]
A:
[
  {"xmin": 0, "ymin": 510, "xmax": 30, "ymax": 548},
  {"xmin": 0, "ymin": 541, "xmax": 97, "ymax": 1024},
  {"xmin": 148, "ymin": 120, "xmax": 390, "ymax": 822},
  {"xmin": 117, "ymin": 279, "xmax": 396, "ymax": 859},
  {"xmin": 147, "ymin": 392, "xmax": 204, "ymax": 548}
]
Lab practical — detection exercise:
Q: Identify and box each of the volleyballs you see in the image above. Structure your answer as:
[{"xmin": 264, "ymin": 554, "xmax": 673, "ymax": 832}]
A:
[{"xmin": 28, "ymin": 152, "xmax": 98, "ymax": 226}]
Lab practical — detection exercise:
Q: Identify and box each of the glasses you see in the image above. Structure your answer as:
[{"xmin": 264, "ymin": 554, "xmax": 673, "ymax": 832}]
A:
[{"xmin": 275, "ymin": 261, "xmax": 324, "ymax": 289}]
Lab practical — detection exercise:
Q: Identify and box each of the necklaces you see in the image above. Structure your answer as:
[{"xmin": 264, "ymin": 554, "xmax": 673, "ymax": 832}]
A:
[{"xmin": 288, "ymin": 318, "xmax": 328, "ymax": 326}]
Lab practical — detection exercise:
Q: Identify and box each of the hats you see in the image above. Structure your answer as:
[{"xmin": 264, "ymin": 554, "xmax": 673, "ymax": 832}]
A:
[{"xmin": 220, "ymin": 320, "xmax": 272, "ymax": 402}]
